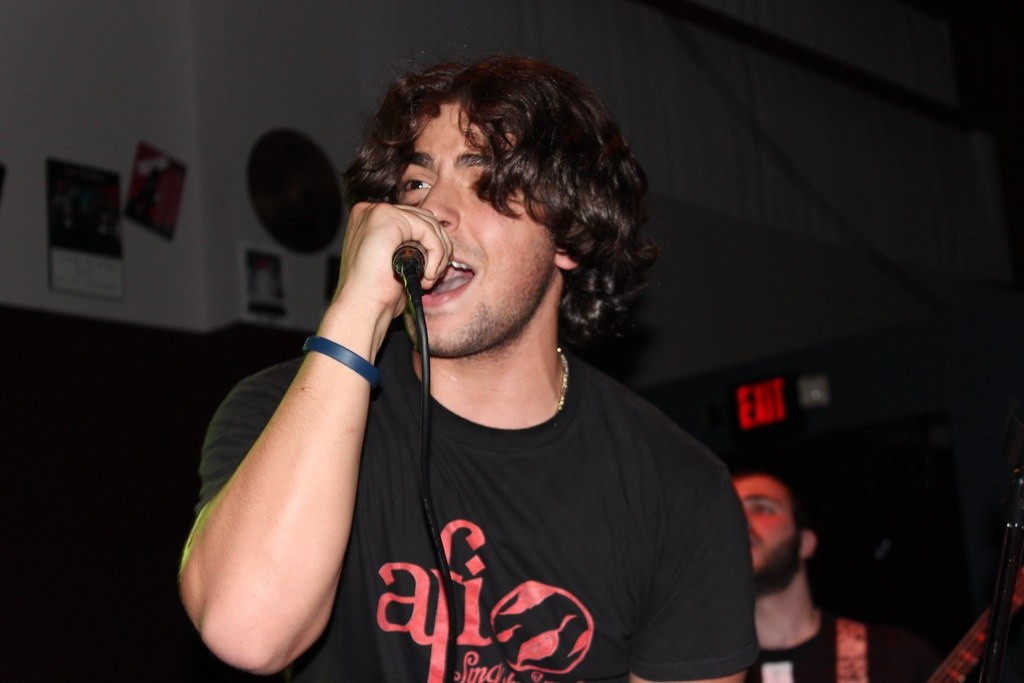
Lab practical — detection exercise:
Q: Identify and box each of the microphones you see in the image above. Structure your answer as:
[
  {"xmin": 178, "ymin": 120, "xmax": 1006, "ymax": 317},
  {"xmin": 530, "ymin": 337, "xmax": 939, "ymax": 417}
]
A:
[{"xmin": 393, "ymin": 239, "xmax": 427, "ymax": 279}]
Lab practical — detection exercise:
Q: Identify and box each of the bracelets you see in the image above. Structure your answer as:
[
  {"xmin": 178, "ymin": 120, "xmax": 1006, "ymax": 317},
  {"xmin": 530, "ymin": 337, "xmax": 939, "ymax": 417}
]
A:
[{"xmin": 303, "ymin": 336, "xmax": 381, "ymax": 388}]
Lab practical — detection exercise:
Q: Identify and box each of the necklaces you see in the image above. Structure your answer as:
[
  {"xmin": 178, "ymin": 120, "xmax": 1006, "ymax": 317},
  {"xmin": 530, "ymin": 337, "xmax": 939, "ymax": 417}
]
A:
[{"xmin": 558, "ymin": 349, "xmax": 568, "ymax": 411}]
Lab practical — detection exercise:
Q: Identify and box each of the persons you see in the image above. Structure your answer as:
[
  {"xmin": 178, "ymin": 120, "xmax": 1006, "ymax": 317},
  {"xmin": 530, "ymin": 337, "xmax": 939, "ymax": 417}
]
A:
[
  {"xmin": 179, "ymin": 54, "xmax": 759, "ymax": 682},
  {"xmin": 729, "ymin": 460, "xmax": 943, "ymax": 683}
]
